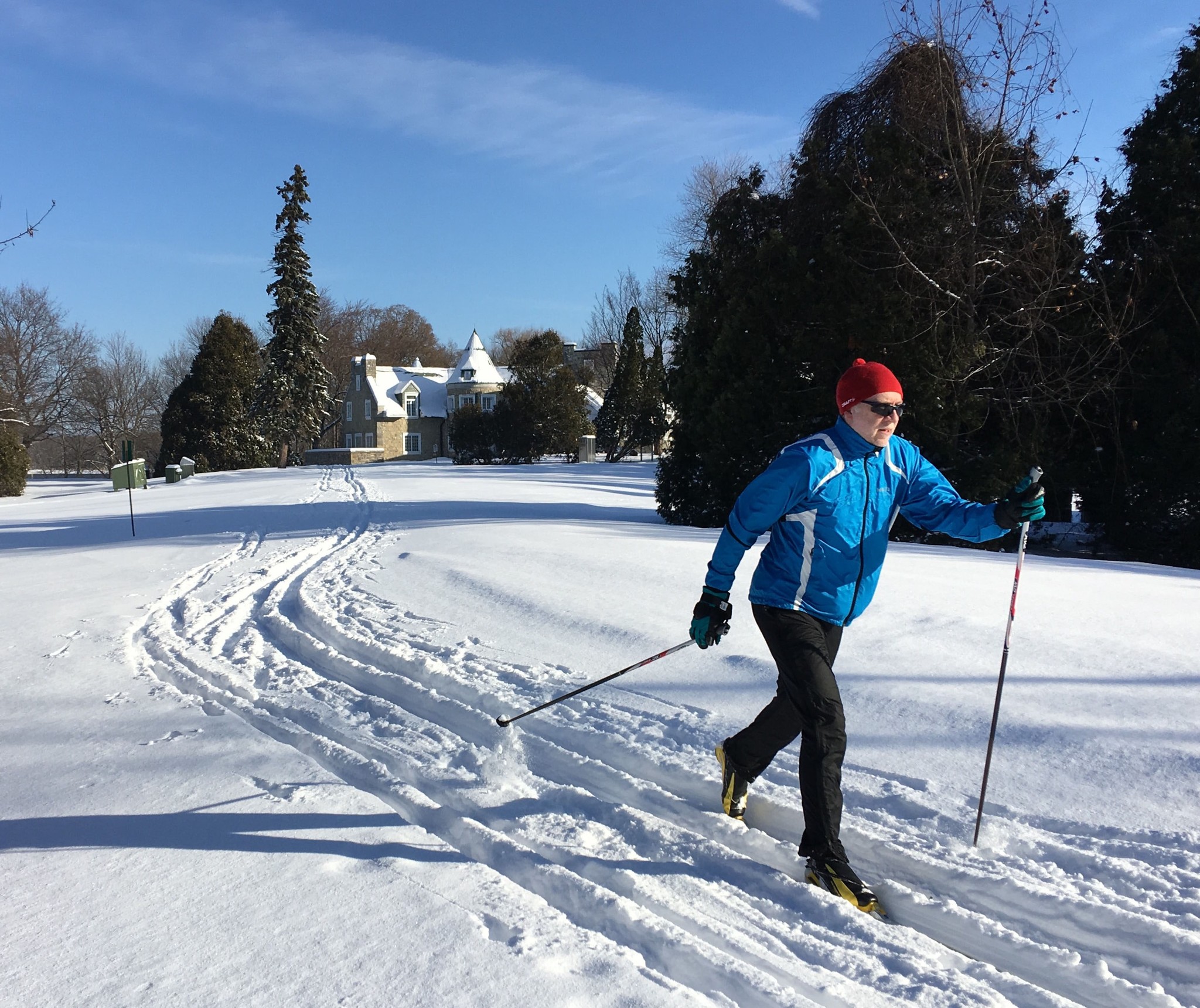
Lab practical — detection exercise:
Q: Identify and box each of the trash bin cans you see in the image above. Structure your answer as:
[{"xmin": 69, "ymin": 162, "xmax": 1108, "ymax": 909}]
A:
[
  {"xmin": 111, "ymin": 458, "xmax": 147, "ymax": 489},
  {"xmin": 180, "ymin": 456, "xmax": 195, "ymax": 479},
  {"xmin": 165, "ymin": 464, "xmax": 183, "ymax": 484}
]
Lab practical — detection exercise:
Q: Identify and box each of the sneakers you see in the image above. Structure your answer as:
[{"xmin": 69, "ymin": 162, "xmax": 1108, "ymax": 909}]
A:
[
  {"xmin": 804, "ymin": 858, "xmax": 878, "ymax": 907},
  {"xmin": 715, "ymin": 740, "xmax": 748, "ymax": 818}
]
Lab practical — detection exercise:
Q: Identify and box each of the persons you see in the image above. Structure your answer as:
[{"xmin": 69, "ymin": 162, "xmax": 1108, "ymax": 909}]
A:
[{"xmin": 689, "ymin": 358, "xmax": 1046, "ymax": 919}]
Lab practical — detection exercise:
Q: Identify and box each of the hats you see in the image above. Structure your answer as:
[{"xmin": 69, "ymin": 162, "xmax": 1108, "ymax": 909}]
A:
[{"xmin": 837, "ymin": 358, "xmax": 903, "ymax": 416}]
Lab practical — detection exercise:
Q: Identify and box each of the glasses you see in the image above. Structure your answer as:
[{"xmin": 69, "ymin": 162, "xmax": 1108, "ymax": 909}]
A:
[{"xmin": 860, "ymin": 400, "xmax": 906, "ymax": 416}]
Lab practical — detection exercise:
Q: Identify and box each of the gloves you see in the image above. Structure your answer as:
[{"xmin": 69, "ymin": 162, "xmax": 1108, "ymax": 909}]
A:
[
  {"xmin": 689, "ymin": 586, "xmax": 732, "ymax": 649},
  {"xmin": 991, "ymin": 476, "xmax": 1045, "ymax": 529}
]
